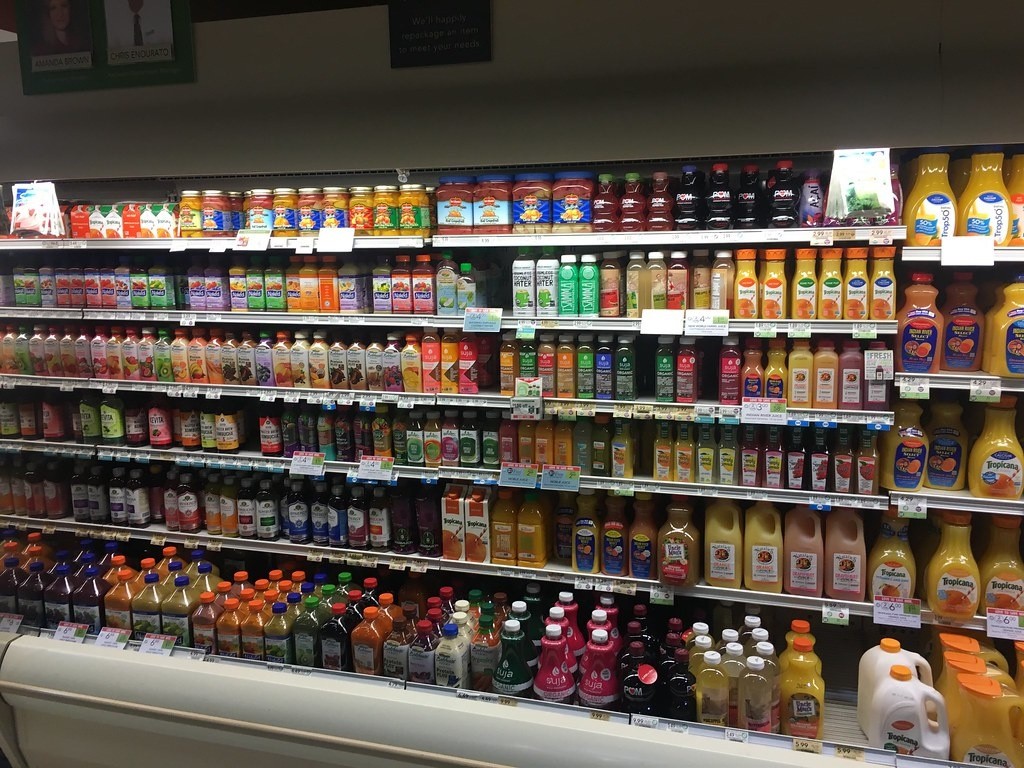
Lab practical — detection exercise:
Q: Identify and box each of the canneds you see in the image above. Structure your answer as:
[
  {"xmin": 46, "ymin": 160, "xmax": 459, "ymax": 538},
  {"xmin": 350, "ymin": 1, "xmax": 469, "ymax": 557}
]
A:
[
  {"xmin": 179, "ymin": 184, "xmax": 438, "ymax": 238},
  {"xmin": 436, "ymin": 170, "xmax": 595, "ymax": 235}
]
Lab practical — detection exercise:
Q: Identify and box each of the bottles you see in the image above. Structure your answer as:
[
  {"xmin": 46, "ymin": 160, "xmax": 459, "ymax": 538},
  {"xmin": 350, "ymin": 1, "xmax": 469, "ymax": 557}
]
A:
[
  {"xmin": 175, "ymin": 254, "xmax": 286, "ymax": 312},
  {"xmin": 286, "ymin": 254, "xmax": 373, "ymax": 314},
  {"xmin": 655, "ymin": 601, "xmax": 825, "ymax": 741},
  {"xmin": 858, "ymin": 626, "xmax": 1024, "ymax": 768},
  {"xmin": 902, "ymin": 145, "xmax": 1024, "ymax": 247},
  {"xmin": 657, "ymin": 495, "xmax": 866, "ymax": 602},
  {"xmin": 880, "ymin": 396, "xmax": 1024, "ymax": 501},
  {"xmin": 0, "ymin": 255, "xmax": 176, "ymax": 309},
  {"xmin": 593, "ymin": 172, "xmax": 673, "ymax": 232},
  {"xmin": 673, "ymin": 163, "xmax": 735, "ymax": 231},
  {"xmin": 655, "ymin": 420, "xmax": 879, "ymax": 495},
  {"xmin": 374, "ymin": 255, "xmax": 392, "ymax": 314},
  {"xmin": 867, "ymin": 506, "xmax": 1024, "ymax": 620},
  {"xmin": 735, "ymin": 160, "xmax": 903, "ymax": 228},
  {"xmin": 895, "ymin": 272, "xmax": 1024, "ymax": 378},
  {"xmin": 656, "ymin": 336, "xmax": 890, "ymax": 410},
  {"xmin": 512, "ymin": 247, "xmax": 895, "ymax": 320},
  {"xmin": 0, "ymin": 323, "xmax": 655, "ymax": 715},
  {"xmin": 392, "ymin": 250, "xmax": 477, "ymax": 316}
]
